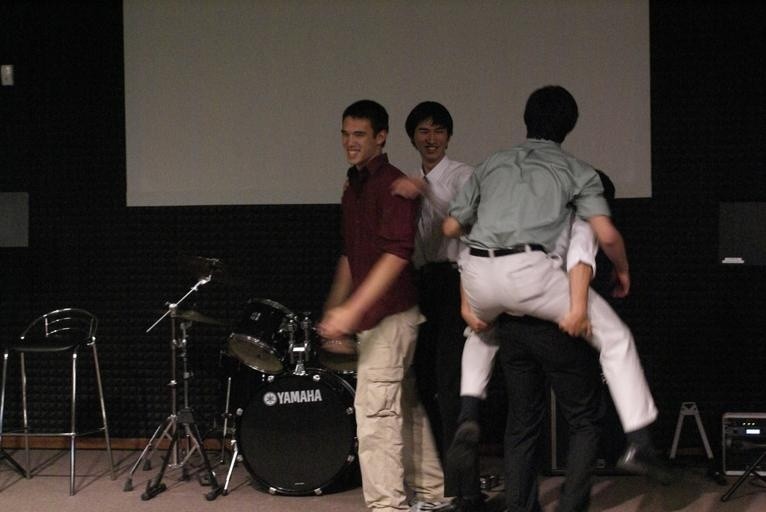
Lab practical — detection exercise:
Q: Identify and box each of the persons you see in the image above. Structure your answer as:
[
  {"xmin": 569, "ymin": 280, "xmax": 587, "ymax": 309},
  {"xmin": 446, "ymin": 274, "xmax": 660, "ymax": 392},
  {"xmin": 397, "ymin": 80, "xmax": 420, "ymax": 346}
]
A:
[
  {"xmin": 390, "ymin": 98, "xmax": 500, "ymax": 512},
  {"xmin": 495, "ymin": 164, "xmax": 618, "ymax": 511},
  {"xmin": 438, "ymin": 82, "xmax": 696, "ymax": 485},
  {"xmin": 313, "ymin": 99, "xmax": 458, "ymax": 512}
]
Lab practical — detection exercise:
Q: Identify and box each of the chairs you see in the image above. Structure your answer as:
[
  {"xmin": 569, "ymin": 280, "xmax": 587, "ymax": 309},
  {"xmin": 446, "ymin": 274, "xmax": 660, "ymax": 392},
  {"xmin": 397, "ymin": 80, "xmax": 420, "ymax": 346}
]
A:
[{"xmin": 1, "ymin": 307, "xmax": 116, "ymax": 494}]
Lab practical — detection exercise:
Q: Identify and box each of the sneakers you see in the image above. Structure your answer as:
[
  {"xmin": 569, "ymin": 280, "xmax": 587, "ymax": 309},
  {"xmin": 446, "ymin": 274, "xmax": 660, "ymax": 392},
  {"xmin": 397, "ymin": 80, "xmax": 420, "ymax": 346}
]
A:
[
  {"xmin": 613, "ymin": 442, "xmax": 686, "ymax": 490},
  {"xmin": 441, "ymin": 417, "xmax": 483, "ymax": 483},
  {"xmin": 411, "ymin": 495, "xmax": 460, "ymax": 512}
]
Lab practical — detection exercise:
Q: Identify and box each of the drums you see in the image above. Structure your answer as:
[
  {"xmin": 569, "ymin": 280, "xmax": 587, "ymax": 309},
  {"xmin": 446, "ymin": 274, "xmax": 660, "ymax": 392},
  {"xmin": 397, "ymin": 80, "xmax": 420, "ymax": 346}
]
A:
[
  {"xmin": 225, "ymin": 296, "xmax": 315, "ymax": 376},
  {"xmin": 234, "ymin": 366, "xmax": 358, "ymax": 489},
  {"xmin": 315, "ymin": 326, "xmax": 360, "ymax": 379}
]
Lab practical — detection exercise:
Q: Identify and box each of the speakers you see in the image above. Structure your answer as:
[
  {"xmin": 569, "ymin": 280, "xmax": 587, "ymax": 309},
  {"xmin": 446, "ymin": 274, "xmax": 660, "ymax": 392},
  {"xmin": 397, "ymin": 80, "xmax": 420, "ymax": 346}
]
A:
[{"xmin": 536, "ymin": 384, "xmax": 626, "ymax": 476}]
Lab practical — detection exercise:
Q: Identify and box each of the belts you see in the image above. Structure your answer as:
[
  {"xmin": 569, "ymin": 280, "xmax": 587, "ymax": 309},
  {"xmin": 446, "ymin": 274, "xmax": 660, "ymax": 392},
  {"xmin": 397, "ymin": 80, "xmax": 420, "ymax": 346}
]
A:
[{"xmin": 467, "ymin": 242, "xmax": 550, "ymax": 260}]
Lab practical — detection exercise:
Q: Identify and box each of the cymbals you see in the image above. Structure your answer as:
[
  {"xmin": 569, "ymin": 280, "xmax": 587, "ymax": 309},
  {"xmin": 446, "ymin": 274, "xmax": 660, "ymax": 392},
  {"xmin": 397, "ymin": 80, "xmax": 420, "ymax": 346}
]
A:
[{"xmin": 169, "ymin": 309, "xmax": 229, "ymax": 326}]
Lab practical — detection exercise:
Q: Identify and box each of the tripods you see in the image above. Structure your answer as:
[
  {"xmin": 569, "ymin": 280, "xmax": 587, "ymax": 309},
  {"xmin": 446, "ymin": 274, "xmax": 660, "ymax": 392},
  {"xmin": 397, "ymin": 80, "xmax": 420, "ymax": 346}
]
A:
[{"xmin": 124, "ymin": 274, "xmax": 239, "ymax": 501}]
